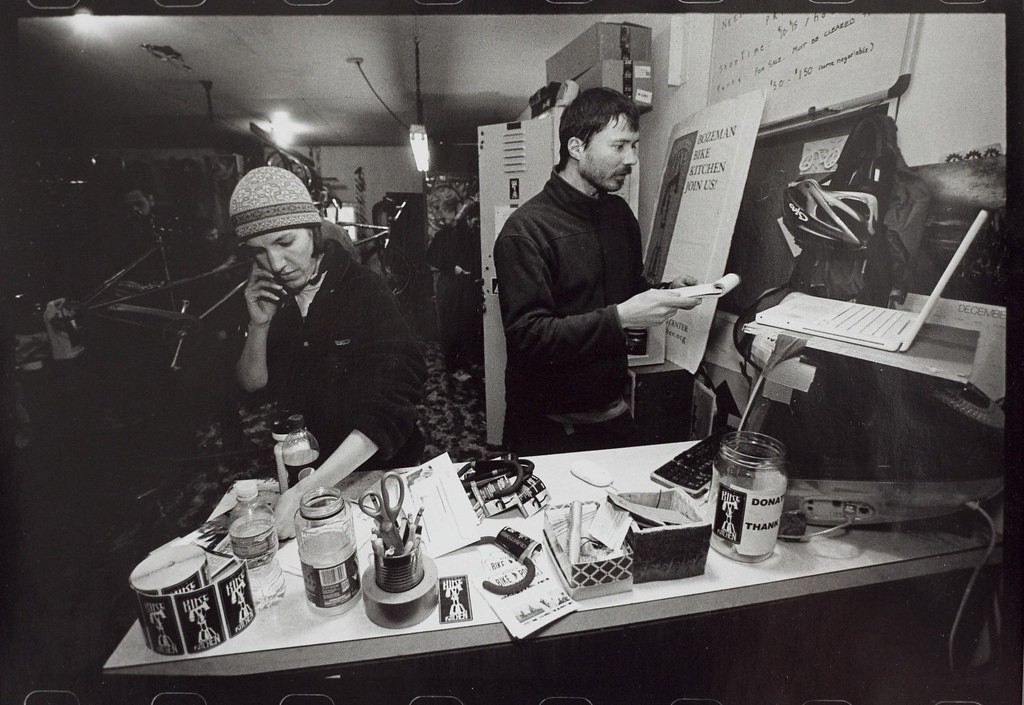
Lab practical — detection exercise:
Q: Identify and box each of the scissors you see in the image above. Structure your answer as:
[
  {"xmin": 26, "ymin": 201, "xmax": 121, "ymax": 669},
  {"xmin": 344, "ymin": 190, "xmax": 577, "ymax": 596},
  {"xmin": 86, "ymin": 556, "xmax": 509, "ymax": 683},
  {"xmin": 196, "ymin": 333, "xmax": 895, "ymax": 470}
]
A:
[{"xmin": 359, "ymin": 472, "xmax": 405, "ymax": 551}]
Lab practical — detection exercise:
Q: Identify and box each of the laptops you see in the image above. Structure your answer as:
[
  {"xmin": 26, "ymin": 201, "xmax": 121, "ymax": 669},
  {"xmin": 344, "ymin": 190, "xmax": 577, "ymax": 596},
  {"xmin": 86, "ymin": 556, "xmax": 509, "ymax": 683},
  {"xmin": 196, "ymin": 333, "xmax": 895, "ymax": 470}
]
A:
[{"xmin": 756, "ymin": 209, "xmax": 988, "ymax": 353}]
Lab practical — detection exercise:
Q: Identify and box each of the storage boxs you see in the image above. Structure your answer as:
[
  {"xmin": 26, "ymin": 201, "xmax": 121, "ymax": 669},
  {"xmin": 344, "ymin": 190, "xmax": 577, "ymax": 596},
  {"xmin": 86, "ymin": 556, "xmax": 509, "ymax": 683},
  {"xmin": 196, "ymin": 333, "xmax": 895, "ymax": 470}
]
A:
[
  {"xmin": 545, "ymin": 21, "xmax": 652, "ymax": 85},
  {"xmin": 576, "ymin": 59, "xmax": 653, "ymax": 113},
  {"xmin": 541, "ymin": 502, "xmax": 634, "ymax": 600},
  {"xmin": 614, "ymin": 485, "xmax": 713, "ymax": 581}
]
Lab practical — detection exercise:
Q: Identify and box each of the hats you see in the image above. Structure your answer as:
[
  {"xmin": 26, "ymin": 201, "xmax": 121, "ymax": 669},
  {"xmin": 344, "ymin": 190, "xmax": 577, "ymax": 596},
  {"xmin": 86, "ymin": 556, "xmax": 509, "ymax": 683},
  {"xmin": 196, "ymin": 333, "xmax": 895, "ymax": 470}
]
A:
[{"xmin": 229, "ymin": 166, "xmax": 322, "ymax": 240}]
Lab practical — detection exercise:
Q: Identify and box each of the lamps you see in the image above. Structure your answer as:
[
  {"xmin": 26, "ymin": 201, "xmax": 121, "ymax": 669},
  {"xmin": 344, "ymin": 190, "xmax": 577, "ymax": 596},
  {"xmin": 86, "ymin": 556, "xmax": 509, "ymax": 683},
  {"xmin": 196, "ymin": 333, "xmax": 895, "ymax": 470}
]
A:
[{"xmin": 408, "ymin": 36, "xmax": 430, "ymax": 172}]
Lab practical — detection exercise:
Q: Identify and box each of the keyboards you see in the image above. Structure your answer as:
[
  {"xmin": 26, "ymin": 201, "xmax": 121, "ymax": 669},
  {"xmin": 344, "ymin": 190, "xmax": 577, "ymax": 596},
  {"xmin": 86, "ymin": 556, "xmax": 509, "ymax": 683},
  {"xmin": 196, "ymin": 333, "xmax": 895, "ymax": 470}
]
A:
[{"xmin": 652, "ymin": 425, "xmax": 739, "ymax": 498}]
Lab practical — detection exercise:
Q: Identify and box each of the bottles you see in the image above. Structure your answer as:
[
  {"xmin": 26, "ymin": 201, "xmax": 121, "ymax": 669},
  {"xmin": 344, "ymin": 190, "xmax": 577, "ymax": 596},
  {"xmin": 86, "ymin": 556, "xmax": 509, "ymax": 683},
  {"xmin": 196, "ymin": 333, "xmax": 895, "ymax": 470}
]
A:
[
  {"xmin": 706, "ymin": 430, "xmax": 788, "ymax": 563},
  {"xmin": 229, "ymin": 480, "xmax": 286, "ymax": 612},
  {"xmin": 272, "ymin": 431, "xmax": 288, "ymax": 495},
  {"xmin": 282, "ymin": 414, "xmax": 321, "ymax": 489},
  {"xmin": 293, "ymin": 488, "xmax": 362, "ymax": 617}
]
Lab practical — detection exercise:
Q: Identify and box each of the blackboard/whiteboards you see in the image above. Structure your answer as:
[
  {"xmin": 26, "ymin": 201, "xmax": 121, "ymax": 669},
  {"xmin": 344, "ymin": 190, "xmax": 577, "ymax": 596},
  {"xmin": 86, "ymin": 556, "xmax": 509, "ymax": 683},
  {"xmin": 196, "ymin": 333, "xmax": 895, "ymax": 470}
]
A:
[{"xmin": 706, "ymin": 12, "xmax": 925, "ymax": 130}]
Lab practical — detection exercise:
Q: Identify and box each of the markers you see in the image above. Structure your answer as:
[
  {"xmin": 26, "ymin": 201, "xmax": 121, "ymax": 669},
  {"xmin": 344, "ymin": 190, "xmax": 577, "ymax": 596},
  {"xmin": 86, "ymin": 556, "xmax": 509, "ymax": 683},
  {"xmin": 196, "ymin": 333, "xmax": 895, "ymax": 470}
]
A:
[{"xmin": 568, "ymin": 499, "xmax": 582, "ymax": 566}]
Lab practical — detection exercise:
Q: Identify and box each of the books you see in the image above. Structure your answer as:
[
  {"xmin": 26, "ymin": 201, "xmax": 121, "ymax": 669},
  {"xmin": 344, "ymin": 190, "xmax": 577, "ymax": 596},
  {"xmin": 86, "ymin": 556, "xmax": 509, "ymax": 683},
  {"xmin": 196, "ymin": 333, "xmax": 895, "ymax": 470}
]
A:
[{"xmin": 652, "ymin": 274, "xmax": 740, "ymax": 298}]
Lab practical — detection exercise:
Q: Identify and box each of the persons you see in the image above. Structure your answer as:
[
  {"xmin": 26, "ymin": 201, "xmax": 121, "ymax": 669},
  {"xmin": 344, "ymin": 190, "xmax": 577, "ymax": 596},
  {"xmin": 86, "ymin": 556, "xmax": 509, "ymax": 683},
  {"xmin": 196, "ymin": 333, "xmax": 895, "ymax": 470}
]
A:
[
  {"xmin": 220, "ymin": 166, "xmax": 426, "ymax": 539},
  {"xmin": 426, "ymin": 202, "xmax": 480, "ymax": 357},
  {"xmin": 492, "ymin": 89, "xmax": 702, "ymax": 454},
  {"xmin": 120, "ymin": 186, "xmax": 187, "ymax": 270}
]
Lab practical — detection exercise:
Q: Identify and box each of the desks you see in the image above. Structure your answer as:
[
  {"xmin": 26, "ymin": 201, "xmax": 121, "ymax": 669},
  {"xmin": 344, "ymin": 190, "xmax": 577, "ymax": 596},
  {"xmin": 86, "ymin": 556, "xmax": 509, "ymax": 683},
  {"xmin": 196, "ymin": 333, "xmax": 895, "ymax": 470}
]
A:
[{"xmin": 104, "ymin": 436, "xmax": 1006, "ymax": 678}]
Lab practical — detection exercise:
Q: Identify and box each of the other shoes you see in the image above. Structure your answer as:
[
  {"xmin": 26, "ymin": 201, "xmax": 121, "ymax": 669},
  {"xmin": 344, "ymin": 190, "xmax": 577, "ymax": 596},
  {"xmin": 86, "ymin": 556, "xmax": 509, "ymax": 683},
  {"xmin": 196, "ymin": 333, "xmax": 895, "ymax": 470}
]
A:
[{"xmin": 451, "ymin": 369, "xmax": 471, "ymax": 382}]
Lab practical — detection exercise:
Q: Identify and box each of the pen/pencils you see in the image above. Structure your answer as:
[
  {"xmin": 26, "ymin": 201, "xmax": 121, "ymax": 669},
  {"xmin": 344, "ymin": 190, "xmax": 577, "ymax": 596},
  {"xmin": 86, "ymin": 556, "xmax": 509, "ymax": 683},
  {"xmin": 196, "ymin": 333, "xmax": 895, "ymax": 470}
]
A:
[{"xmin": 370, "ymin": 506, "xmax": 426, "ymax": 556}]
